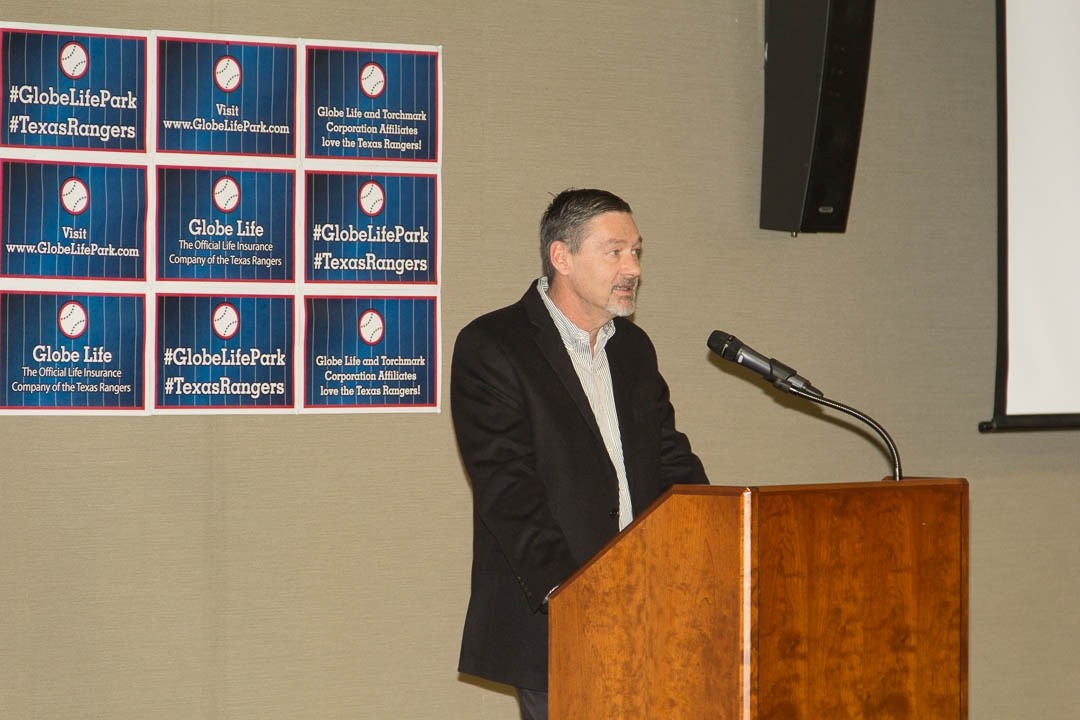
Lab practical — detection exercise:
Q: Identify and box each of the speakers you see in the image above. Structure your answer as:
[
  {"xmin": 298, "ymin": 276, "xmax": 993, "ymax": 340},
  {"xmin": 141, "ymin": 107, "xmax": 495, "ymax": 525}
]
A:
[{"xmin": 760, "ymin": 0, "xmax": 876, "ymax": 235}]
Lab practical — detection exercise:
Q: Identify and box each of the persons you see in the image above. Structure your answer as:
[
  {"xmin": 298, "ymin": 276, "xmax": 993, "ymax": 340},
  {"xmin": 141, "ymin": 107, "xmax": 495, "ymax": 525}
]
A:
[{"xmin": 450, "ymin": 189, "xmax": 712, "ymax": 720}]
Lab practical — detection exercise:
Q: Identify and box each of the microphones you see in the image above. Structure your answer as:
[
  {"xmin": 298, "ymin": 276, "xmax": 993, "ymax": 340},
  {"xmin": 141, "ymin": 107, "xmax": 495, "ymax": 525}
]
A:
[{"xmin": 706, "ymin": 330, "xmax": 823, "ymax": 398}]
